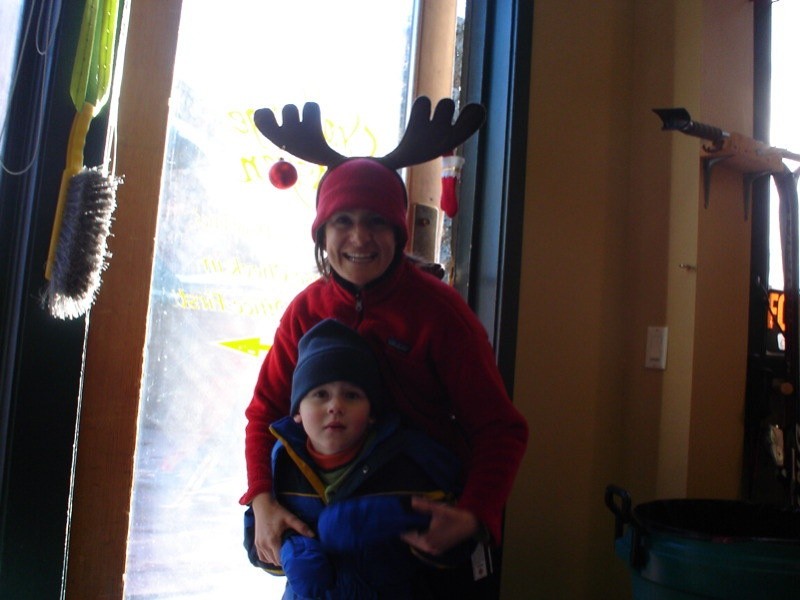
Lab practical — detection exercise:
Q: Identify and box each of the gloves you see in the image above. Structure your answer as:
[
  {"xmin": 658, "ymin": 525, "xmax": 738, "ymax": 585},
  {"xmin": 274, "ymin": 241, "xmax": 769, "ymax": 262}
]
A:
[
  {"xmin": 279, "ymin": 535, "xmax": 338, "ymax": 599},
  {"xmin": 316, "ymin": 493, "xmax": 421, "ymax": 562}
]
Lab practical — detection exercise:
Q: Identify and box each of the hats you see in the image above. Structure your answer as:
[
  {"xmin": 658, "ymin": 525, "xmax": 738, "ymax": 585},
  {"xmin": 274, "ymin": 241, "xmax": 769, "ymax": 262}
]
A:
[
  {"xmin": 288, "ymin": 318, "xmax": 379, "ymax": 417},
  {"xmin": 311, "ymin": 158, "xmax": 407, "ymax": 251}
]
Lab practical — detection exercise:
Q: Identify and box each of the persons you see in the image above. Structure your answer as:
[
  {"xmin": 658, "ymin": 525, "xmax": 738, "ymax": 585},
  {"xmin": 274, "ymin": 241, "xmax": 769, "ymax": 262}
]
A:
[
  {"xmin": 238, "ymin": 96, "xmax": 528, "ymax": 567},
  {"xmin": 244, "ymin": 320, "xmax": 500, "ymax": 600}
]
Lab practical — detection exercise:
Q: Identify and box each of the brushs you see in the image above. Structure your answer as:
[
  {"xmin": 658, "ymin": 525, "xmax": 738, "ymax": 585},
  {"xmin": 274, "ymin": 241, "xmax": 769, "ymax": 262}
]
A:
[{"xmin": 45, "ymin": 103, "xmax": 117, "ymax": 318}]
